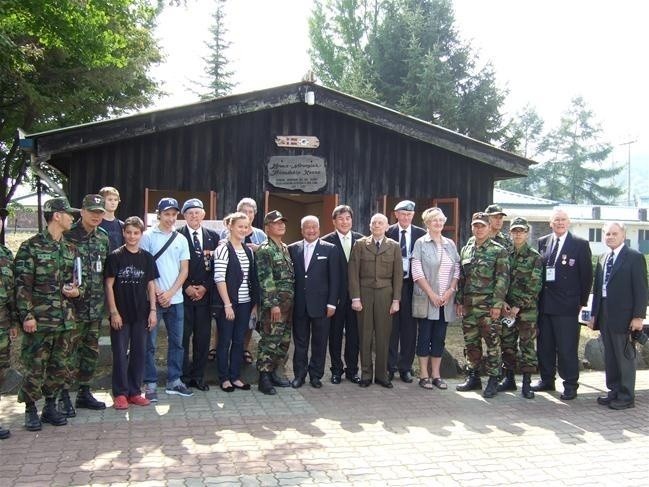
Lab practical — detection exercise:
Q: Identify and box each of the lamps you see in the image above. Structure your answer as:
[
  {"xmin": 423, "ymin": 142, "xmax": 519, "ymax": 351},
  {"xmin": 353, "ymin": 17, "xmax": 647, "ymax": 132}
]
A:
[{"xmin": 302, "ymin": 71, "xmax": 318, "ymax": 106}]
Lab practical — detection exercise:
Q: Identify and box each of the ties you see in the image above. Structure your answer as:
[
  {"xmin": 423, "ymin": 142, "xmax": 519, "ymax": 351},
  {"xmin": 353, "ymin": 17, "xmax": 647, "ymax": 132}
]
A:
[
  {"xmin": 375, "ymin": 242, "xmax": 379, "ymax": 251},
  {"xmin": 400, "ymin": 230, "xmax": 406, "ymax": 258},
  {"xmin": 343, "ymin": 235, "xmax": 350, "ymax": 263},
  {"xmin": 548, "ymin": 239, "xmax": 560, "ymax": 266},
  {"xmin": 605, "ymin": 251, "xmax": 614, "ymax": 283},
  {"xmin": 305, "ymin": 244, "xmax": 312, "ymax": 273},
  {"xmin": 192, "ymin": 231, "xmax": 202, "ymax": 259}
]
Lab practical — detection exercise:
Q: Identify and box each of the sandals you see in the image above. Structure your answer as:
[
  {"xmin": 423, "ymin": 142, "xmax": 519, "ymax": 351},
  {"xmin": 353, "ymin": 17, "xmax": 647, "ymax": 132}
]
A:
[
  {"xmin": 242, "ymin": 350, "xmax": 253, "ymax": 364},
  {"xmin": 207, "ymin": 349, "xmax": 218, "ymax": 361}
]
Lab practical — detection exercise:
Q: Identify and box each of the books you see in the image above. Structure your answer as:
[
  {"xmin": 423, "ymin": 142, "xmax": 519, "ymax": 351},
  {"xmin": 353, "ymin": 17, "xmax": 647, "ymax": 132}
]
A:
[{"xmin": 578, "ymin": 305, "xmax": 592, "ymax": 325}]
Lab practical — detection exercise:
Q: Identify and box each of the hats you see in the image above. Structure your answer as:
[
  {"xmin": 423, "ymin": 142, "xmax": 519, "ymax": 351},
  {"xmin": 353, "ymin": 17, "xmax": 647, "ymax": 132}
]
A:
[
  {"xmin": 264, "ymin": 210, "xmax": 288, "ymax": 224},
  {"xmin": 394, "ymin": 200, "xmax": 415, "ymax": 211},
  {"xmin": 471, "ymin": 205, "xmax": 529, "ymax": 231},
  {"xmin": 43, "ymin": 194, "xmax": 205, "ymax": 213}
]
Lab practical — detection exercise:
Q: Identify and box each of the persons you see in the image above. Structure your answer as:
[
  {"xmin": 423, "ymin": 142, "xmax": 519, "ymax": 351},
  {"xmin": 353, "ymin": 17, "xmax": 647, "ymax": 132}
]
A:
[
  {"xmin": 0, "ymin": 185, "xmax": 296, "ymax": 438},
  {"xmin": 529, "ymin": 208, "xmax": 593, "ymax": 401},
  {"xmin": 586, "ymin": 220, "xmax": 649, "ymax": 410},
  {"xmin": 288, "ymin": 200, "xmax": 543, "ymax": 397}
]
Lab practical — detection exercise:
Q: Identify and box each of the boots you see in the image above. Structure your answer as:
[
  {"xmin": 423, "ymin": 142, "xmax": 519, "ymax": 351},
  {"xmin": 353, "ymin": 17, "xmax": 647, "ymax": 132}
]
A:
[
  {"xmin": 456, "ymin": 370, "xmax": 635, "ymax": 409},
  {"xmin": 0, "ymin": 384, "xmax": 105, "ymax": 440}
]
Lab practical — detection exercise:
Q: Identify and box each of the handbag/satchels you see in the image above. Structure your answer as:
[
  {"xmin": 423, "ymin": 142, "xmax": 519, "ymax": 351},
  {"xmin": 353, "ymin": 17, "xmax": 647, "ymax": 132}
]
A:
[
  {"xmin": 249, "ymin": 314, "xmax": 256, "ymax": 330},
  {"xmin": 411, "ymin": 296, "xmax": 428, "ymax": 319}
]
